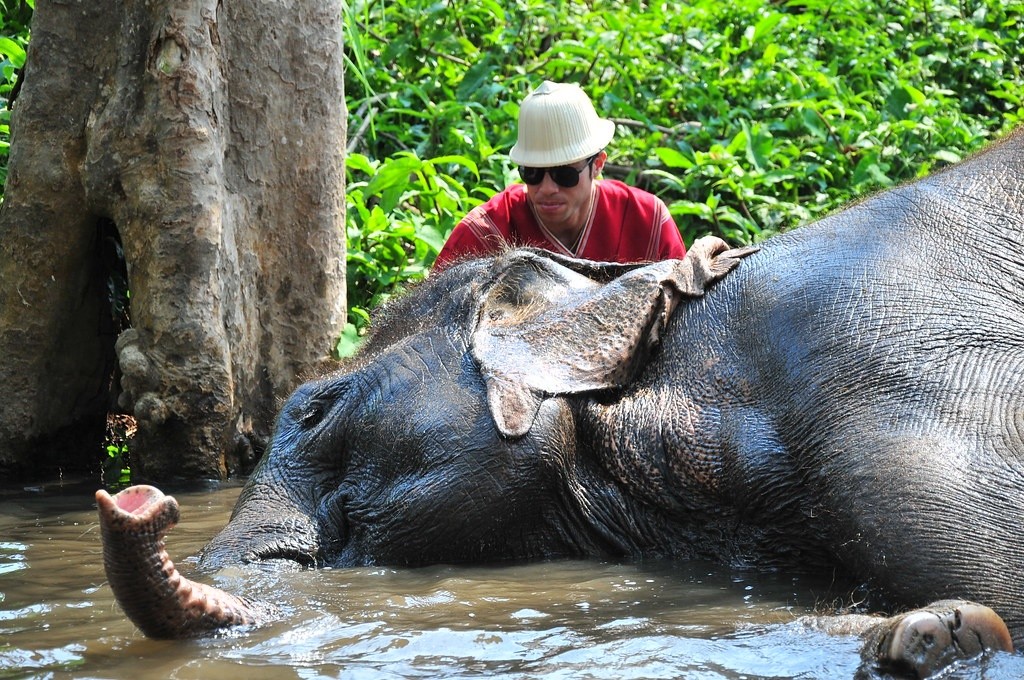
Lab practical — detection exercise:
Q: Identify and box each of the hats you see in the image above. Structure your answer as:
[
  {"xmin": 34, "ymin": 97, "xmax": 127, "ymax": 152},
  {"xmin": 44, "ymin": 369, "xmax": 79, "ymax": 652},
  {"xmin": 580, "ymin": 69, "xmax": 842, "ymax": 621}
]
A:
[{"xmin": 510, "ymin": 80, "xmax": 615, "ymax": 167}]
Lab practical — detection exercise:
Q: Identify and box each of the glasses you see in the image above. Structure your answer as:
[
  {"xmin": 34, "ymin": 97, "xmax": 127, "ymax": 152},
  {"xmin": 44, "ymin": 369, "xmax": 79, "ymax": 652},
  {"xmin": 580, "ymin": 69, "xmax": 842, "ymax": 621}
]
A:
[{"xmin": 518, "ymin": 157, "xmax": 593, "ymax": 188}]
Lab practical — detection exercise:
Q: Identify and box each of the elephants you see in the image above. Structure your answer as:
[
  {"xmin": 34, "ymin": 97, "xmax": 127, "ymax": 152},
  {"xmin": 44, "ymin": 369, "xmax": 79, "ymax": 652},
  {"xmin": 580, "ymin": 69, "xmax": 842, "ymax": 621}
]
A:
[{"xmin": 90, "ymin": 124, "xmax": 1022, "ymax": 680}]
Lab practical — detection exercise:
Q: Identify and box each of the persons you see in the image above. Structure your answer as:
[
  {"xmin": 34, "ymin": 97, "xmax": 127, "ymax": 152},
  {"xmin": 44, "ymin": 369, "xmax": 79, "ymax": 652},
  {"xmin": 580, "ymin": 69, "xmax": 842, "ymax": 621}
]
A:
[{"xmin": 431, "ymin": 79, "xmax": 687, "ymax": 275}]
